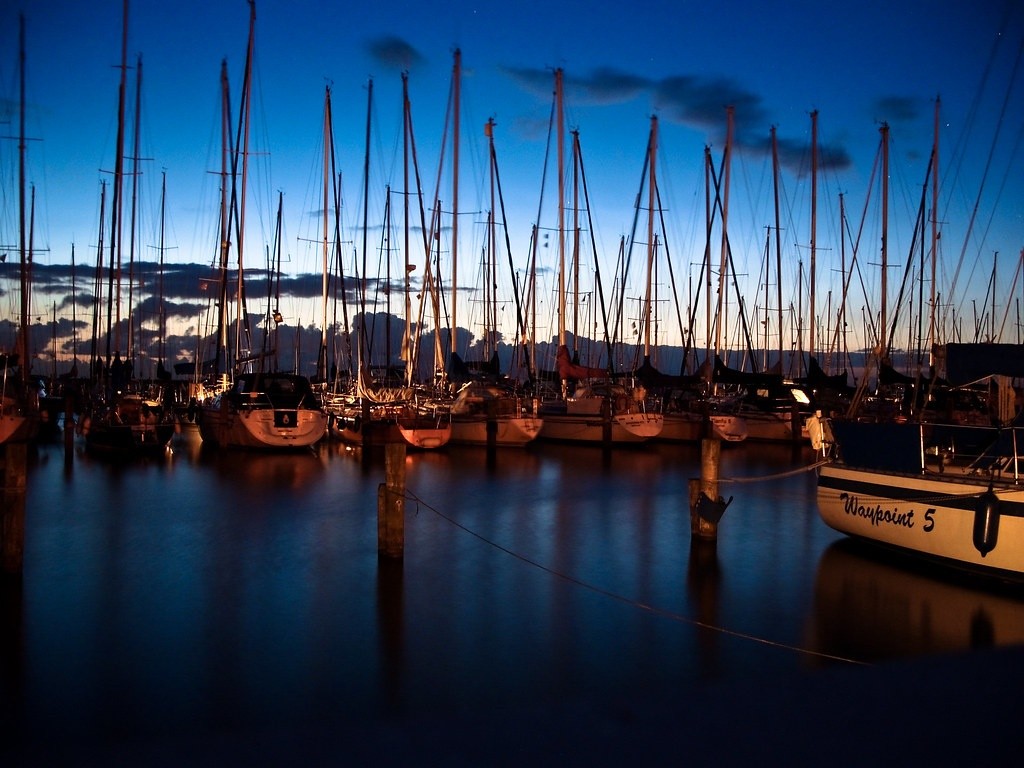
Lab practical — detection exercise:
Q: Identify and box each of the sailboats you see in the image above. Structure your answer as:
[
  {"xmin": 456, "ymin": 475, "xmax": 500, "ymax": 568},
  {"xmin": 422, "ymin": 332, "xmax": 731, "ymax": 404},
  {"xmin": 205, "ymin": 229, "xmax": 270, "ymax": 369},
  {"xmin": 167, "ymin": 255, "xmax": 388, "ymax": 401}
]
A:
[{"xmin": 0, "ymin": 1, "xmax": 1024, "ymax": 574}]
